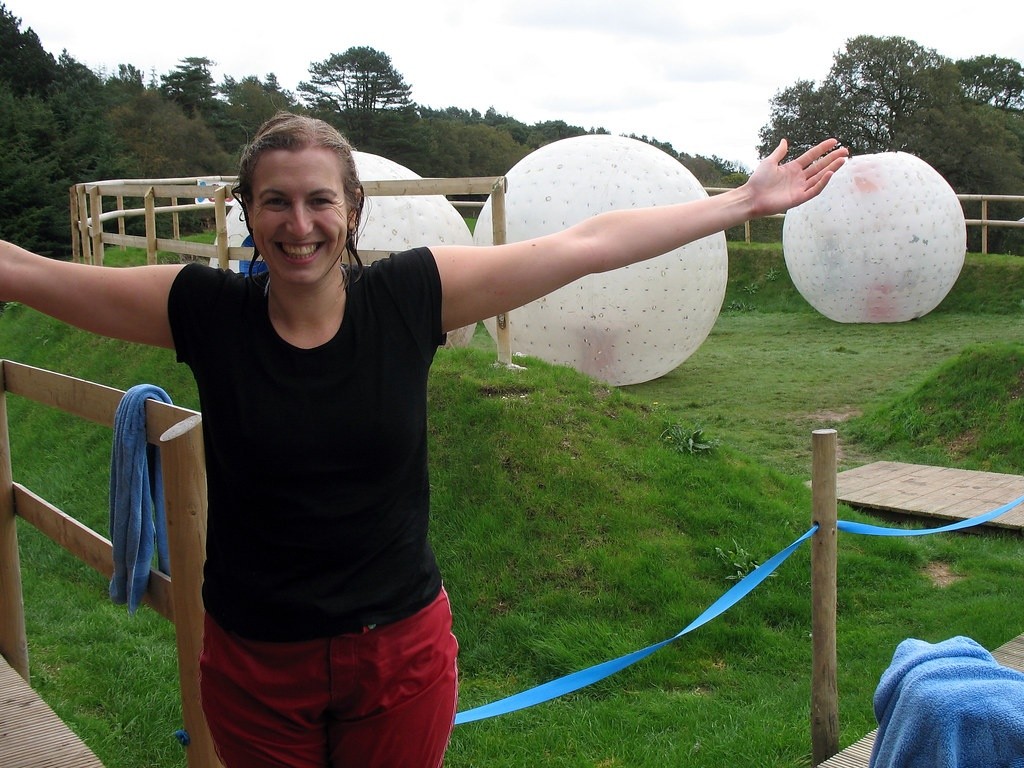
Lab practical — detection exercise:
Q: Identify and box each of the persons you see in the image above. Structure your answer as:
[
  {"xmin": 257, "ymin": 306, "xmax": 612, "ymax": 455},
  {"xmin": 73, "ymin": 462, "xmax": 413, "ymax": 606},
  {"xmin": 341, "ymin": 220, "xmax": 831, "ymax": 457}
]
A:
[{"xmin": 0, "ymin": 111, "xmax": 849, "ymax": 768}]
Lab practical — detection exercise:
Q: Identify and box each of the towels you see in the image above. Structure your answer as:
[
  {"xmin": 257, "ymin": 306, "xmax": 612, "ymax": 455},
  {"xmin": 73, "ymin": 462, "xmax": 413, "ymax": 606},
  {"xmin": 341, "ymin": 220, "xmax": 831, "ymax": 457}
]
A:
[
  {"xmin": 107, "ymin": 383, "xmax": 173, "ymax": 613},
  {"xmin": 867, "ymin": 633, "xmax": 1024, "ymax": 767}
]
